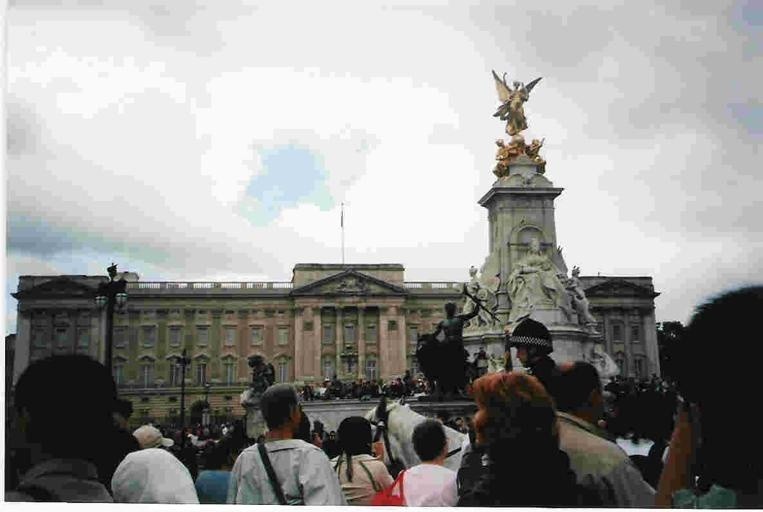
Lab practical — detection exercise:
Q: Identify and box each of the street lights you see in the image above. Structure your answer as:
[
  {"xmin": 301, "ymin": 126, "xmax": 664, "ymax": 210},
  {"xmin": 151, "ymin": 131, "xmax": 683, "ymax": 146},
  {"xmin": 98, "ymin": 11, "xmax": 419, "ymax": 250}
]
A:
[
  {"xmin": 202, "ymin": 382, "xmax": 212, "ymax": 430},
  {"xmin": 174, "ymin": 347, "xmax": 189, "ymax": 454},
  {"xmin": 94, "ymin": 264, "xmax": 127, "ymax": 373},
  {"xmin": 339, "ymin": 343, "xmax": 359, "ymax": 373},
  {"xmin": 223, "ymin": 394, "xmax": 234, "ymax": 428}
]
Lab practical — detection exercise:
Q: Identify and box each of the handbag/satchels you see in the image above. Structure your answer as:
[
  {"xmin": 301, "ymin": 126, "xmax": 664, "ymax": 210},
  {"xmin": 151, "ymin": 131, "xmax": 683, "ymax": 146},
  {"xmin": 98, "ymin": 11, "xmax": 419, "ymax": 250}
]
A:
[{"xmin": 371, "ymin": 472, "xmax": 405, "ymax": 504}]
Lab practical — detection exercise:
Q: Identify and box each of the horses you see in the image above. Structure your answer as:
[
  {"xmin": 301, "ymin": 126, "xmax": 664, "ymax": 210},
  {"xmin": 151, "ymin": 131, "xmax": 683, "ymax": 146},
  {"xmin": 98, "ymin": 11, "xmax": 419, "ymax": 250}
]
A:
[{"xmin": 363, "ymin": 393, "xmax": 467, "ymax": 472}]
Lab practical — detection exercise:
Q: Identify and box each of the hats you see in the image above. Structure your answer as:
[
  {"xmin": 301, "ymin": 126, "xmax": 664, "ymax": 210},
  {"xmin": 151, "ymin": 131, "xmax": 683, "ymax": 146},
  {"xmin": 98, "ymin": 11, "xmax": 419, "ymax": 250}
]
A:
[
  {"xmin": 132, "ymin": 425, "xmax": 174, "ymax": 448},
  {"xmin": 110, "ymin": 449, "xmax": 199, "ymax": 503},
  {"xmin": 508, "ymin": 318, "xmax": 553, "ymax": 352}
]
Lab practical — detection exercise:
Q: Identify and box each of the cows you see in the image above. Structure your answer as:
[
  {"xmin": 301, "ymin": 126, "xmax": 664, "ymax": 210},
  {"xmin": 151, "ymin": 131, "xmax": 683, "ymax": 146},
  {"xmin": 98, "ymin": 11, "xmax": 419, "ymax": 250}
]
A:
[{"xmin": 414, "ymin": 328, "xmax": 464, "ymax": 389}]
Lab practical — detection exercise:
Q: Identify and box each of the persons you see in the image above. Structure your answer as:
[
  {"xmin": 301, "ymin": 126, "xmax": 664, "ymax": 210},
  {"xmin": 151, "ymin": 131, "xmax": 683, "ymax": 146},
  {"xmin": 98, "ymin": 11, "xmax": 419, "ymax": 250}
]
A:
[
  {"xmin": 493, "ymin": 69, "xmax": 544, "ymax": 135},
  {"xmin": 493, "ymin": 135, "xmax": 546, "ymax": 176},
  {"xmin": 510, "ymin": 232, "xmax": 573, "ymax": 328}
]
